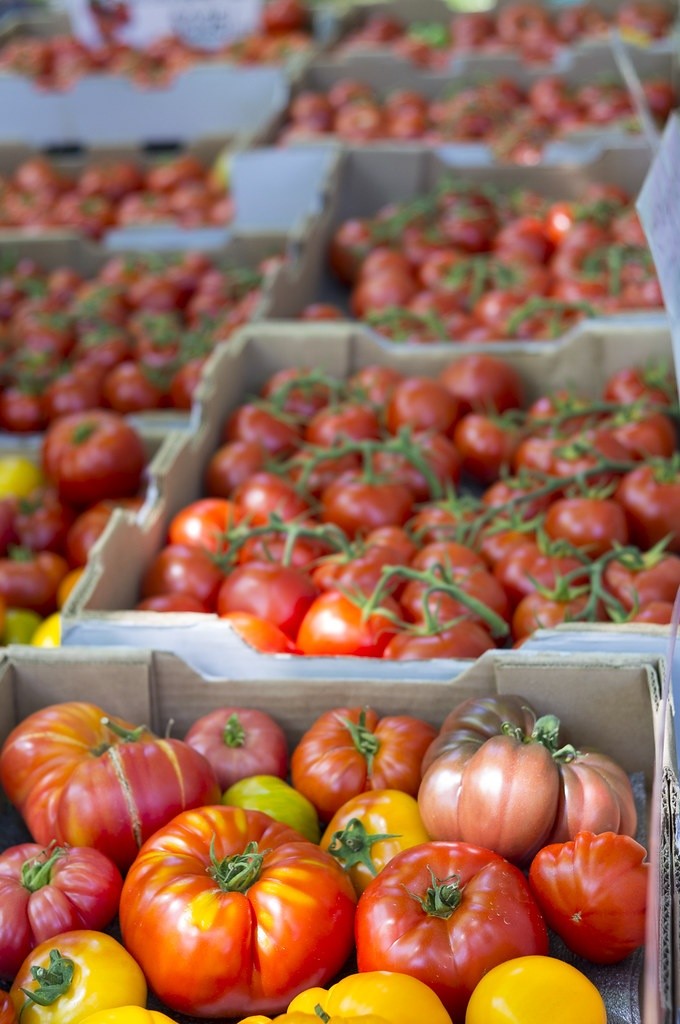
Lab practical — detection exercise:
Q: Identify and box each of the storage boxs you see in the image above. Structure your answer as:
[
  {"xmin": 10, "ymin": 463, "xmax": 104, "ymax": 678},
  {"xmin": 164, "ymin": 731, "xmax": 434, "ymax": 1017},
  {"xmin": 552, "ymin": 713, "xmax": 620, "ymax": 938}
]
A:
[
  {"xmin": 0, "ymin": 228, "xmax": 296, "ymax": 445},
  {"xmin": 0, "ymin": 0, "xmax": 680, "ymax": 245},
  {"xmin": 252, "ymin": 142, "xmax": 680, "ymax": 380},
  {"xmin": 0, "ymin": 651, "xmax": 680, "ymax": 1024},
  {"xmin": 59, "ymin": 324, "xmax": 680, "ymax": 777}
]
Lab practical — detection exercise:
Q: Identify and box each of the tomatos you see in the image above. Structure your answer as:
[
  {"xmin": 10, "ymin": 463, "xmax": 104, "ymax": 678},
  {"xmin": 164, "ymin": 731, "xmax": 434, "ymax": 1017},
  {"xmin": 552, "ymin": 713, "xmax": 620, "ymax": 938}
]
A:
[{"xmin": 0, "ymin": 0, "xmax": 680, "ymax": 1024}]
